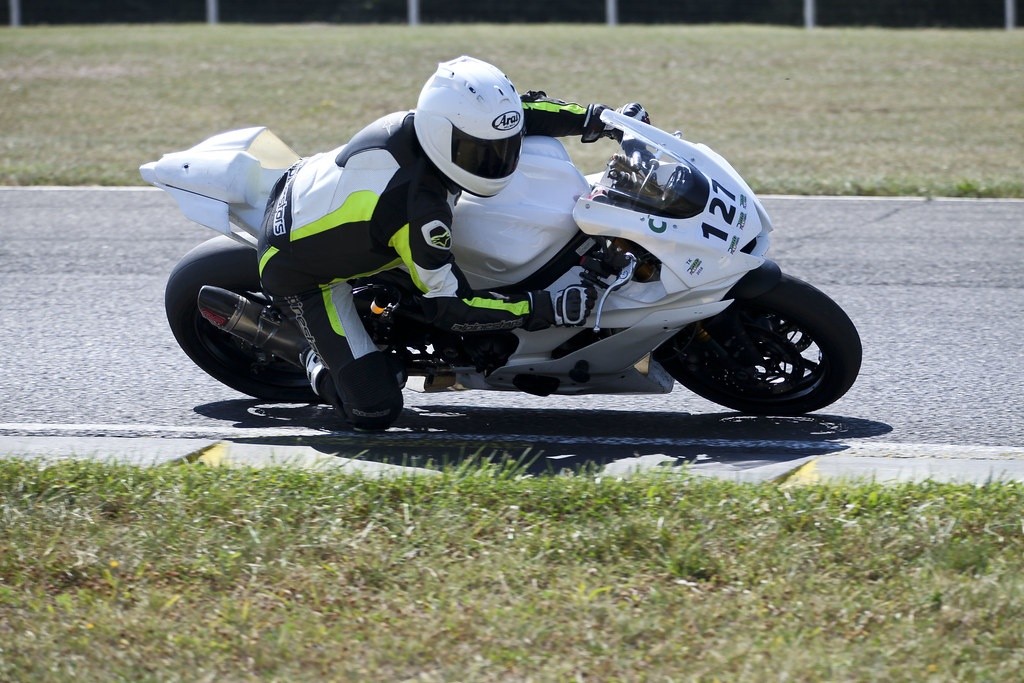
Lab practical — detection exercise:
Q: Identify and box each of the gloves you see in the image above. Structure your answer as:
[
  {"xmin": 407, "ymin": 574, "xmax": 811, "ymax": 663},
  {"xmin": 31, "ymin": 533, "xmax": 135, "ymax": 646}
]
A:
[
  {"xmin": 520, "ymin": 284, "xmax": 598, "ymax": 333},
  {"xmin": 581, "ymin": 102, "xmax": 650, "ymax": 145}
]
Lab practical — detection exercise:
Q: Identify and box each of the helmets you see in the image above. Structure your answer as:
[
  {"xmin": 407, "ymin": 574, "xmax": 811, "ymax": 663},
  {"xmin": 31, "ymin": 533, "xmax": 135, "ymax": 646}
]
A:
[{"xmin": 414, "ymin": 56, "xmax": 524, "ymax": 198}]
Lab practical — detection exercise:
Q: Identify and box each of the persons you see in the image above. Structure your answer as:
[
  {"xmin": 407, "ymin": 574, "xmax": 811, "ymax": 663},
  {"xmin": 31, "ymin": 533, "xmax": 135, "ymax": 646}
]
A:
[{"xmin": 259, "ymin": 54, "xmax": 650, "ymax": 436}]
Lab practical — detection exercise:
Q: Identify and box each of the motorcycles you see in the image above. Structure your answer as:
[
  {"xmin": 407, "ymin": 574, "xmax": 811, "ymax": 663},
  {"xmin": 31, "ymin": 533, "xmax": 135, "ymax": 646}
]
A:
[{"xmin": 139, "ymin": 105, "xmax": 864, "ymax": 418}]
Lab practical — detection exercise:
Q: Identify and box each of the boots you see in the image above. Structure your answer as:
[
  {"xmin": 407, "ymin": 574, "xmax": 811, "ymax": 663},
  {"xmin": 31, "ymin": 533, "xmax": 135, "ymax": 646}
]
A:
[{"xmin": 300, "ymin": 347, "xmax": 330, "ymax": 396}]
